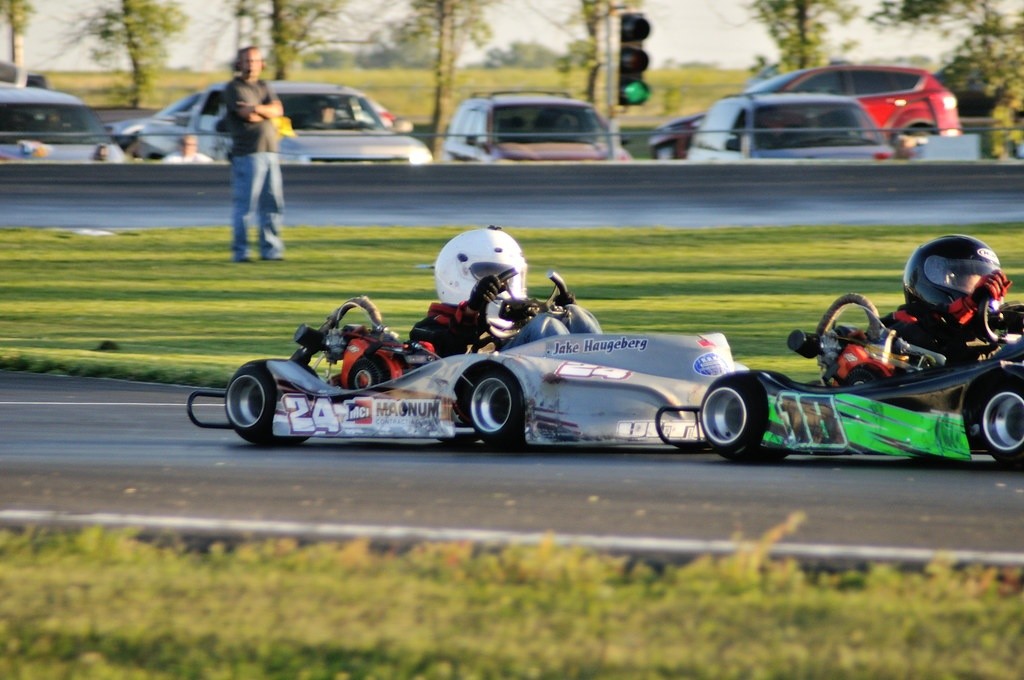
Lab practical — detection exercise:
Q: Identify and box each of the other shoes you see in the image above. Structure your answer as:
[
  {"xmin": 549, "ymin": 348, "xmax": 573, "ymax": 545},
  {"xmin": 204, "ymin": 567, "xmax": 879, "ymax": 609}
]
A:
[
  {"xmin": 233, "ymin": 252, "xmax": 255, "ymax": 264},
  {"xmin": 261, "ymin": 252, "xmax": 284, "ymax": 260}
]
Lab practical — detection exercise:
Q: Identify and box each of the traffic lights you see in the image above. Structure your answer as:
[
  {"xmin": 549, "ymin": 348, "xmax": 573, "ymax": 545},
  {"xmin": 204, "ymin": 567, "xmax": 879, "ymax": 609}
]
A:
[{"xmin": 605, "ymin": 10, "xmax": 653, "ymax": 108}]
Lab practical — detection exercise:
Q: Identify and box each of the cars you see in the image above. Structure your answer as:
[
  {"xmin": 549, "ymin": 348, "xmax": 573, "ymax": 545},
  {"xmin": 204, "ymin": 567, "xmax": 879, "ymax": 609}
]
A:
[
  {"xmin": 441, "ymin": 87, "xmax": 635, "ymax": 161},
  {"xmin": 648, "ymin": 64, "xmax": 963, "ymax": 161},
  {"xmin": 0, "ymin": 83, "xmax": 116, "ymax": 163},
  {"xmin": 104, "ymin": 80, "xmax": 436, "ymax": 164},
  {"xmin": 687, "ymin": 93, "xmax": 897, "ymax": 159}
]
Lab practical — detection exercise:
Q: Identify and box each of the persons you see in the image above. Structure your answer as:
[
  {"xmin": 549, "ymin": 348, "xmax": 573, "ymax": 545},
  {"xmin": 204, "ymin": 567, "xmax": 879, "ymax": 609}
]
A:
[
  {"xmin": 303, "ymin": 98, "xmax": 337, "ymax": 129},
  {"xmin": 879, "ymin": 234, "xmax": 1011, "ymax": 369},
  {"xmin": 409, "ymin": 229, "xmax": 602, "ymax": 358},
  {"xmin": 885, "ymin": 136, "xmax": 919, "ymax": 161},
  {"xmin": 90, "ymin": 135, "xmax": 214, "ymax": 164},
  {"xmin": 226, "ymin": 44, "xmax": 289, "ymax": 261}
]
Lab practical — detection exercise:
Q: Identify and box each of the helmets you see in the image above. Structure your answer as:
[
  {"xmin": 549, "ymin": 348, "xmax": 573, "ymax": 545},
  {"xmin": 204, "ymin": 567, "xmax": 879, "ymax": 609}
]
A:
[
  {"xmin": 902, "ymin": 235, "xmax": 1002, "ymax": 327},
  {"xmin": 434, "ymin": 229, "xmax": 527, "ymax": 305}
]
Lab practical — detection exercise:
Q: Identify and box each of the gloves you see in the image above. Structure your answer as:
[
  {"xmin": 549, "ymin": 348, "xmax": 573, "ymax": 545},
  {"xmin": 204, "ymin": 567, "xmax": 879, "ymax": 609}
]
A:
[
  {"xmin": 466, "ymin": 274, "xmax": 507, "ymax": 312},
  {"xmin": 554, "ymin": 292, "xmax": 576, "ymax": 310},
  {"xmin": 964, "ymin": 269, "xmax": 1013, "ymax": 311}
]
